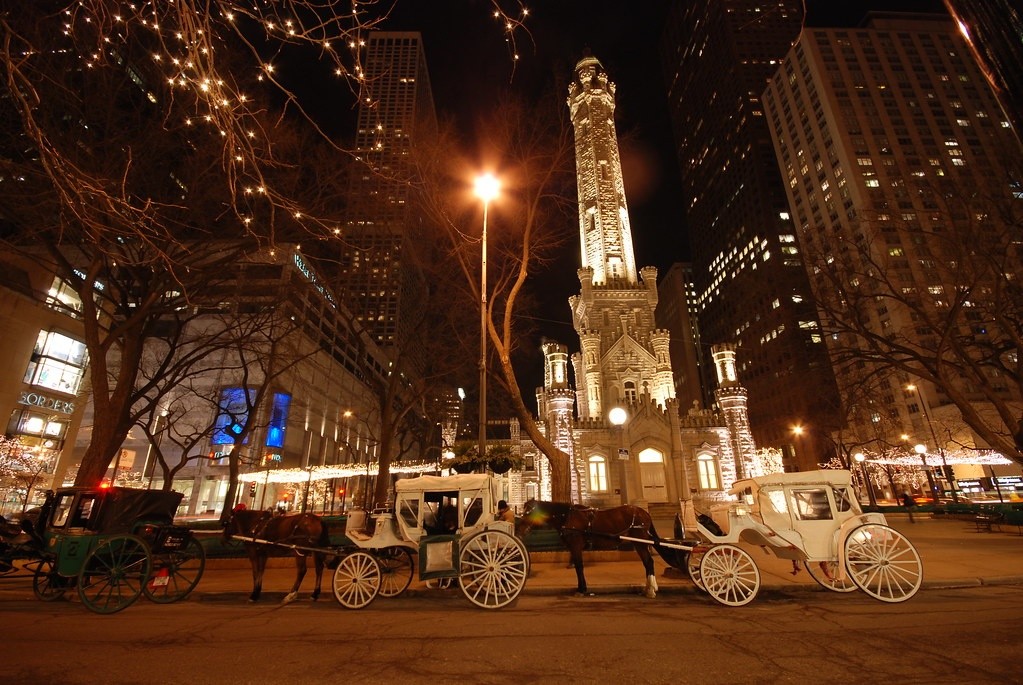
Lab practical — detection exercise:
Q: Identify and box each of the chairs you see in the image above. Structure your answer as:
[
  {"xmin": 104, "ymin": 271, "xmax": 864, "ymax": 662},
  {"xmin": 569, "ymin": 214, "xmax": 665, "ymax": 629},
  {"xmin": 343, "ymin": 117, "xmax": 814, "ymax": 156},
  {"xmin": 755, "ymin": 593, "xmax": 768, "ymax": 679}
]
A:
[{"xmin": 976, "ymin": 510, "xmax": 1004, "ymax": 533}]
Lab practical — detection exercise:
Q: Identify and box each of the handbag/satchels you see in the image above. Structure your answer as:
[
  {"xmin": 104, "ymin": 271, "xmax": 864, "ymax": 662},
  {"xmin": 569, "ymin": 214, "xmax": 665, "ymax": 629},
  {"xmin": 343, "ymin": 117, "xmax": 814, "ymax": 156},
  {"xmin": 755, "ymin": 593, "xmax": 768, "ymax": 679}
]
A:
[{"xmin": 695, "ymin": 509, "xmax": 723, "ymax": 536}]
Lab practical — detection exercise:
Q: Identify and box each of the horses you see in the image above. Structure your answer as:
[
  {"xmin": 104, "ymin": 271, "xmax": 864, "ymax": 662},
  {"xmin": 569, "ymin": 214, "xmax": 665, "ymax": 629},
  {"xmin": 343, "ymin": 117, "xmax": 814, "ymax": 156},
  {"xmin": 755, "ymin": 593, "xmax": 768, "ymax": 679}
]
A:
[
  {"xmin": 518, "ymin": 497, "xmax": 659, "ymax": 599},
  {"xmin": 223, "ymin": 509, "xmax": 327, "ymax": 606}
]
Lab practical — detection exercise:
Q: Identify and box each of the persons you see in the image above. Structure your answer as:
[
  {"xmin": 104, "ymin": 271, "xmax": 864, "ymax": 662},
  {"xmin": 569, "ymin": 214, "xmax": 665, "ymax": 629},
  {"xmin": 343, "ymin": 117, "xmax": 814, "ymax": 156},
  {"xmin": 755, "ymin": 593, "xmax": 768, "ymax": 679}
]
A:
[
  {"xmin": 903, "ymin": 494, "xmax": 915, "ymax": 523},
  {"xmin": 494, "ymin": 500, "xmax": 516, "ymax": 524},
  {"xmin": 35, "ymin": 490, "xmax": 55, "ymax": 533}
]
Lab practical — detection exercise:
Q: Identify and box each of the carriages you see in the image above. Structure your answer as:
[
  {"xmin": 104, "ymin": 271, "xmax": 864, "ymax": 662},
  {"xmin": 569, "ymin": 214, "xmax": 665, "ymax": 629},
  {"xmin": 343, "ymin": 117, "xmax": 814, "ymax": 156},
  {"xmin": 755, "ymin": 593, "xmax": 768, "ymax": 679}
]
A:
[
  {"xmin": 0, "ymin": 482, "xmax": 209, "ymax": 616},
  {"xmin": 218, "ymin": 470, "xmax": 531, "ymax": 608},
  {"xmin": 512, "ymin": 469, "xmax": 925, "ymax": 606}
]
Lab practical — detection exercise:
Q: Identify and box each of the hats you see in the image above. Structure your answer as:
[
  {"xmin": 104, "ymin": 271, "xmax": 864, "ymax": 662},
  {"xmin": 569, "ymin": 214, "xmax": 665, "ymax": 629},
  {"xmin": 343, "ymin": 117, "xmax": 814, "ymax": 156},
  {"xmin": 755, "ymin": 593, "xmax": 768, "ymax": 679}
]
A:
[{"xmin": 498, "ymin": 500, "xmax": 506, "ymax": 508}]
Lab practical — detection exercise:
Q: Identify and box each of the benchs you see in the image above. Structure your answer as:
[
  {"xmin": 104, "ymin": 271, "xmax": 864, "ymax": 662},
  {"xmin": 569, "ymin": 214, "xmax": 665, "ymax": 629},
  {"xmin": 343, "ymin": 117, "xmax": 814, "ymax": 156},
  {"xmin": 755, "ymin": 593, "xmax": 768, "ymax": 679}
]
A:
[
  {"xmin": 709, "ymin": 499, "xmax": 747, "ymax": 532},
  {"xmin": 371, "ymin": 501, "xmax": 396, "ymax": 520}
]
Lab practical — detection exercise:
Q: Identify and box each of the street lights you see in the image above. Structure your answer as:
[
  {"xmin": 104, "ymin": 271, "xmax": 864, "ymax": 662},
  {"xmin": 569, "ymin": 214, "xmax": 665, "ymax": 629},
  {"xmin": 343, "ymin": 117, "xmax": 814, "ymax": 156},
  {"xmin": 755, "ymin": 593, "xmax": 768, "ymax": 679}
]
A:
[
  {"xmin": 478, "ymin": 172, "xmax": 505, "ymax": 469},
  {"xmin": 906, "ymin": 382, "xmax": 959, "ymax": 504},
  {"xmin": 855, "ymin": 453, "xmax": 881, "ymax": 513},
  {"xmin": 915, "ymin": 443, "xmax": 941, "ymax": 506}
]
